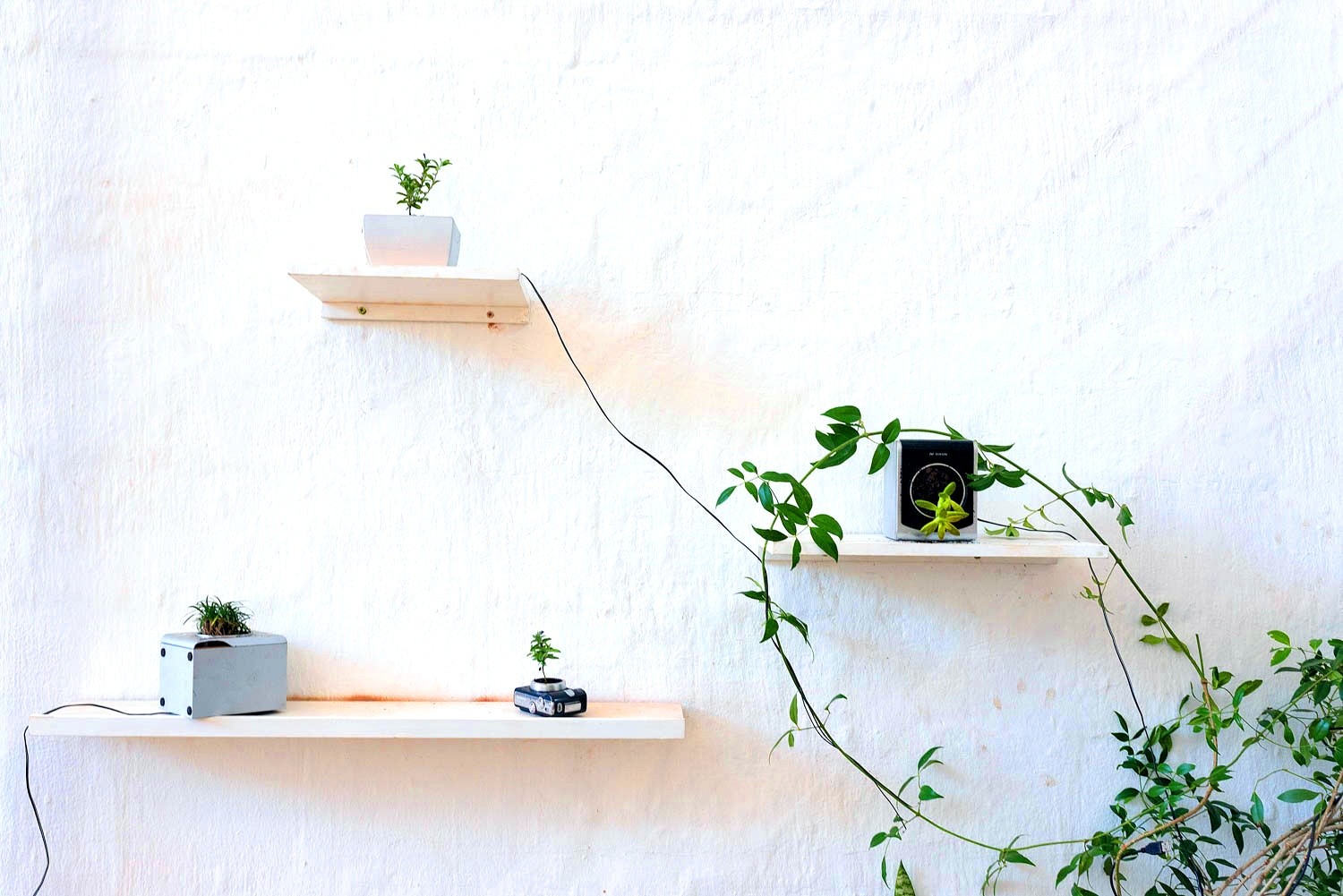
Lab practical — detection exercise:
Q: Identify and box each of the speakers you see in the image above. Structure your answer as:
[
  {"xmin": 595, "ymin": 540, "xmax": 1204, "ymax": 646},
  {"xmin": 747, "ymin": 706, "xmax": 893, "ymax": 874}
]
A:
[{"xmin": 881, "ymin": 436, "xmax": 977, "ymax": 541}]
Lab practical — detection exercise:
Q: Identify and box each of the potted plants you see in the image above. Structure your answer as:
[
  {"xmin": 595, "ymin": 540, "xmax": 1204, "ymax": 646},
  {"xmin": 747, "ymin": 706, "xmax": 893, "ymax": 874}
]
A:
[
  {"xmin": 363, "ymin": 149, "xmax": 462, "ymax": 269},
  {"xmin": 514, "ymin": 630, "xmax": 587, "ymax": 717},
  {"xmin": 158, "ymin": 595, "xmax": 290, "ymax": 719}
]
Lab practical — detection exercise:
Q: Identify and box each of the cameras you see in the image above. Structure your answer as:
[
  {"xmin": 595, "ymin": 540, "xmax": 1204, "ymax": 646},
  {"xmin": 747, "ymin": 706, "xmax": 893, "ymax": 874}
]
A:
[{"xmin": 513, "ymin": 678, "xmax": 587, "ymax": 716}]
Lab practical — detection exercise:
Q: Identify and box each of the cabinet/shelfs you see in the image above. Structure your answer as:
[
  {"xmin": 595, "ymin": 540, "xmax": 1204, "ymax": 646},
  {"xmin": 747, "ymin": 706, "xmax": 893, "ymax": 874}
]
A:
[{"xmin": 34, "ymin": 271, "xmax": 1108, "ymax": 739}]
